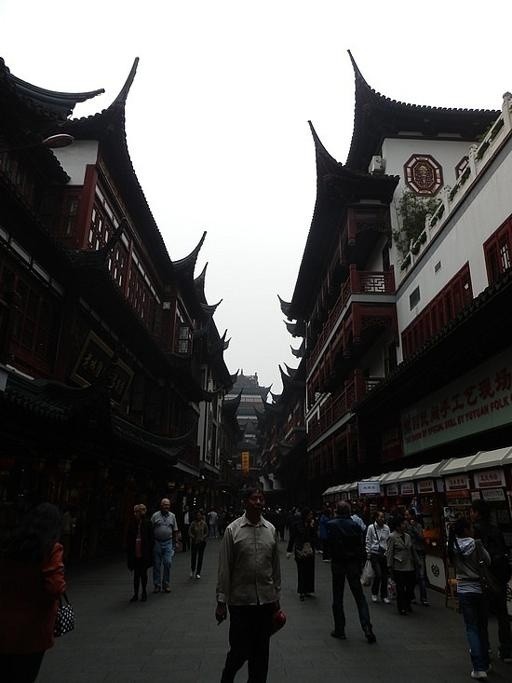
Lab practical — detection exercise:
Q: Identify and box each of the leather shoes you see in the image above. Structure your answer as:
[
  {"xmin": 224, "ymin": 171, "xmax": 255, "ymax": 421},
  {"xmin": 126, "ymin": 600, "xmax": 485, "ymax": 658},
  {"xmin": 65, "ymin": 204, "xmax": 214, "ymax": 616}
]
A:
[{"xmin": 331, "ymin": 631, "xmax": 346, "ymax": 639}]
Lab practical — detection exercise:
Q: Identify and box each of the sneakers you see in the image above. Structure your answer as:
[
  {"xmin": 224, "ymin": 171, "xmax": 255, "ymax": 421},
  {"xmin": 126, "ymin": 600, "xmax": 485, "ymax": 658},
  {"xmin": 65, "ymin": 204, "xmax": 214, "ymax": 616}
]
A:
[
  {"xmin": 154, "ymin": 586, "xmax": 171, "ymax": 593},
  {"xmin": 372, "ymin": 595, "xmax": 390, "ymax": 603},
  {"xmin": 471, "ymin": 671, "xmax": 487, "ymax": 678}
]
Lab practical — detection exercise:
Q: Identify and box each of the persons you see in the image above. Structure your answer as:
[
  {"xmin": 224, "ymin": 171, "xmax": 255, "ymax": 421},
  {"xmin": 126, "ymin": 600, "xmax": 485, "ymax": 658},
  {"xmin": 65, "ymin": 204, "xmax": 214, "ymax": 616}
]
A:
[
  {"xmin": 127, "ymin": 488, "xmax": 510, "ymax": 681},
  {"xmin": 0, "ymin": 447, "xmax": 80, "ymax": 683},
  {"xmin": 314, "ymin": 385, "xmax": 321, "ymax": 402}
]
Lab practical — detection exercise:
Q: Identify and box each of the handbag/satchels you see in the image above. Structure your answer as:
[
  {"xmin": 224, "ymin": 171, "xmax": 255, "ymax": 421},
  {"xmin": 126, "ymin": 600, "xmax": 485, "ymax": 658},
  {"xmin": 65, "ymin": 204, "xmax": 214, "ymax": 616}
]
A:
[
  {"xmin": 54, "ymin": 592, "xmax": 74, "ymax": 637},
  {"xmin": 296, "ymin": 542, "xmax": 314, "ymax": 559}
]
